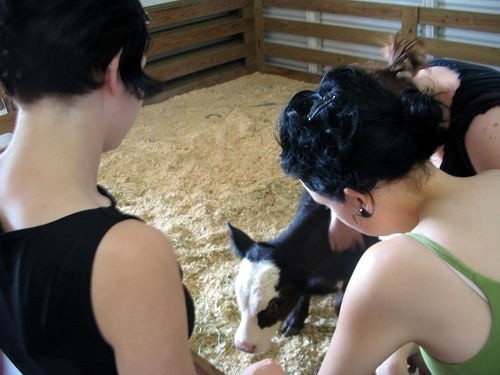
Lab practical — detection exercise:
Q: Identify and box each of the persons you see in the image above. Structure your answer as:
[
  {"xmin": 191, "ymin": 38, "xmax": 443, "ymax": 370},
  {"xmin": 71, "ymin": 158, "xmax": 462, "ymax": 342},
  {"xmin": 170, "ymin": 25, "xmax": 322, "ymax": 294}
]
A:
[
  {"xmin": 0, "ymin": 0, "xmax": 198, "ymax": 375},
  {"xmin": 273, "ymin": 31, "xmax": 500, "ymax": 375}
]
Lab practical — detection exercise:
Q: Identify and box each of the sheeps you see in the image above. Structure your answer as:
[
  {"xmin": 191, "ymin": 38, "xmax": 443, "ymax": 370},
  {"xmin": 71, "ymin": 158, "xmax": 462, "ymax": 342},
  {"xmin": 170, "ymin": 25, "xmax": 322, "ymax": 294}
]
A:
[{"xmin": 227, "ymin": 188, "xmax": 382, "ymax": 354}]
